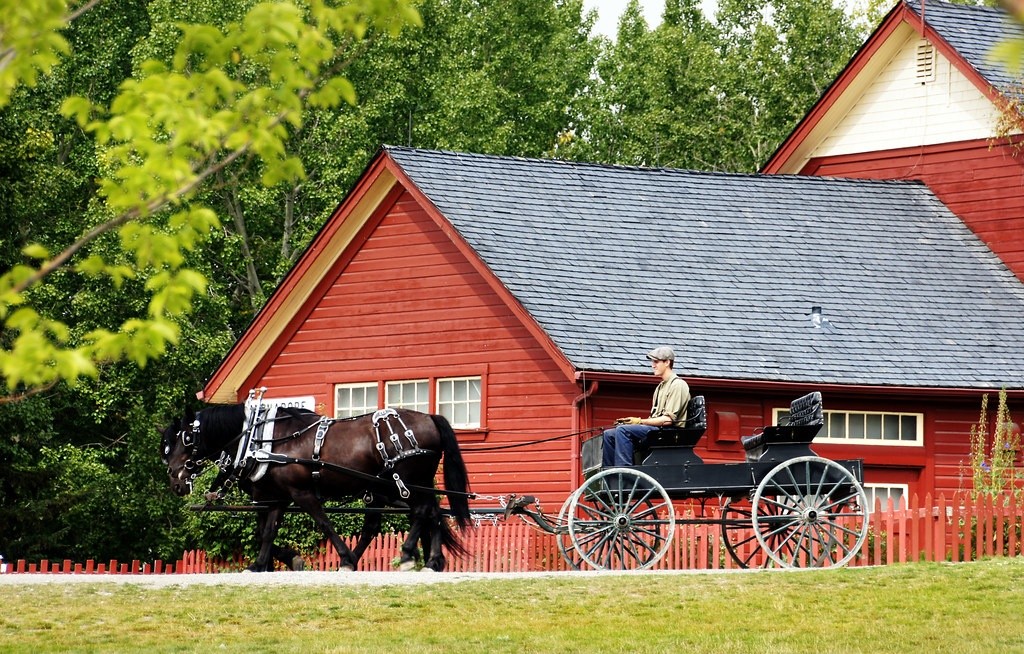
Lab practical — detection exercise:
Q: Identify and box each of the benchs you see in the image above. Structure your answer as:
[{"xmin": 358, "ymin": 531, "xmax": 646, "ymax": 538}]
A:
[
  {"xmin": 740, "ymin": 392, "xmax": 824, "ymax": 450},
  {"xmin": 639, "ymin": 395, "xmax": 706, "ymax": 447}
]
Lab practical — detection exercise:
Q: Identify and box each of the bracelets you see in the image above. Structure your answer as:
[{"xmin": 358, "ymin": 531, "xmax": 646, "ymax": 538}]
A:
[{"xmin": 637, "ymin": 417, "xmax": 641, "ymax": 424}]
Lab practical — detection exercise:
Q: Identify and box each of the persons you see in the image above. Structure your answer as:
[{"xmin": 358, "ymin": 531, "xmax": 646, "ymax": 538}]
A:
[{"xmin": 602, "ymin": 347, "xmax": 691, "ymax": 466}]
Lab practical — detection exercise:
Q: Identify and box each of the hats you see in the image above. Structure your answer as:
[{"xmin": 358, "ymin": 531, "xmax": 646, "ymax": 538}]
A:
[{"xmin": 645, "ymin": 347, "xmax": 674, "ymax": 361}]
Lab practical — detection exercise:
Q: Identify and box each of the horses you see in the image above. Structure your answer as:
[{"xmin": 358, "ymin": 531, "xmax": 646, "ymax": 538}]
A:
[{"xmin": 159, "ymin": 393, "xmax": 471, "ymax": 573}]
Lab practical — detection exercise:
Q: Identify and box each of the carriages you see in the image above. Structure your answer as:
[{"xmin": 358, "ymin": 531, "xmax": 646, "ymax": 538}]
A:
[{"xmin": 163, "ymin": 385, "xmax": 869, "ymax": 572}]
